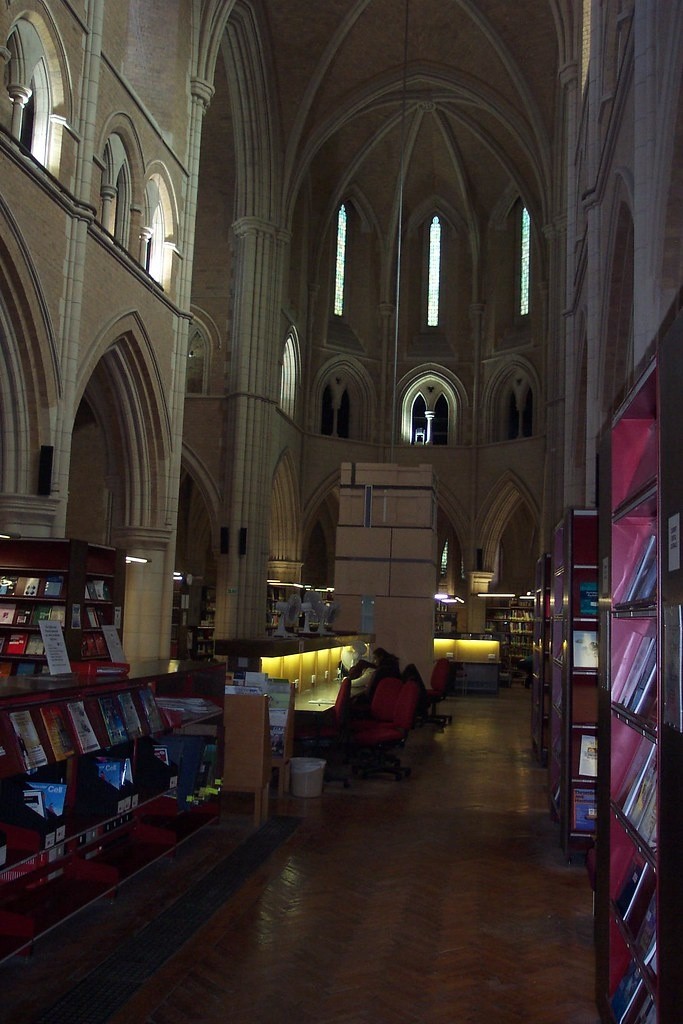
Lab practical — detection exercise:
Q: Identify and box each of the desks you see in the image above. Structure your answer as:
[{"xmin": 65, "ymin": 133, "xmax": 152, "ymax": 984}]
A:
[{"xmin": 214, "ymin": 634, "xmax": 374, "ymax": 753}]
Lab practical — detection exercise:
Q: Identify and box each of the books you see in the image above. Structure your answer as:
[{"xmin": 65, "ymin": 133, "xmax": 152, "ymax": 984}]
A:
[
  {"xmin": 32, "ymin": 603, "xmax": 66, "ymax": 627},
  {"xmin": 615, "ymin": 850, "xmax": 657, "ymax": 976},
  {"xmin": 578, "ymin": 734, "xmax": 598, "ymax": 777},
  {"xmin": 571, "ymin": 787, "xmax": 597, "ymax": 834},
  {"xmin": 93, "ymin": 756, "xmax": 133, "ymax": 790},
  {"xmin": 97, "ymin": 694, "xmax": 128, "ymax": 746},
  {"xmin": 573, "ymin": 630, "xmax": 599, "ymax": 671},
  {"xmin": 137, "ymin": 687, "xmax": 166, "ymax": 733},
  {"xmin": 117, "ymin": 692, "xmax": 145, "ymax": 740},
  {"xmin": 611, "ymin": 631, "xmax": 657, "ymax": 714},
  {"xmin": 619, "ymin": 534, "xmax": 656, "ymax": 603},
  {"xmin": 579, "ymin": 581, "xmax": 598, "ymax": 615},
  {"xmin": 83, "ymin": 607, "xmax": 107, "ymax": 628},
  {"xmin": 0, "ymin": 575, "xmax": 18, "ymax": 595},
  {"xmin": 6, "ymin": 632, "xmax": 44, "ymax": 655},
  {"xmin": 269, "ymin": 707, "xmax": 288, "ymax": 759},
  {"xmin": 81, "ymin": 631, "xmax": 108, "ymax": 657},
  {"xmin": 0, "ymin": 661, "xmax": 12, "ymax": 678},
  {"xmin": 610, "ymin": 958, "xmax": 656, "ymax": 1024},
  {"xmin": 485, "ymin": 599, "xmax": 534, "ymax": 659},
  {"xmin": 621, "ymin": 742, "xmax": 658, "ymax": 857},
  {"xmin": 9, "ymin": 710, "xmax": 48, "ymax": 770},
  {"xmin": 0, "ymin": 636, "xmax": 6, "ymax": 654},
  {"xmin": 15, "ymin": 576, "xmax": 40, "ymax": 597},
  {"xmin": 84, "ymin": 579, "xmax": 113, "ymax": 602},
  {"xmin": 16, "ymin": 604, "xmax": 32, "ymax": 624},
  {"xmin": 64, "ymin": 699, "xmax": 102, "ymax": 755},
  {"xmin": 38, "ymin": 661, "xmax": 49, "ymax": 674},
  {"xmin": 44, "ymin": 575, "xmax": 64, "ymax": 598},
  {"xmin": 40, "ymin": 705, "xmax": 77, "ymax": 762},
  {"xmin": 153, "ymin": 744, "xmax": 169, "ymax": 767},
  {"xmin": 435, "ymin": 602, "xmax": 448, "ymax": 631},
  {"xmin": 16, "ymin": 661, "xmax": 35, "ymax": 675},
  {"xmin": 22, "ymin": 781, "xmax": 67, "ymax": 821},
  {"xmin": 0, "ymin": 604, "xmax": 16, "ymax": 625}
]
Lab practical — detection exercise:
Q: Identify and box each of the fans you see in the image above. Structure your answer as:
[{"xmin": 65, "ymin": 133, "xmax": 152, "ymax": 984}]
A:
[
  {"xmin": 316, "ymin": 600, "xmax": 342, "ymax": 635},
  {"xmin": 300, "ymin": 590, "xmax": 324, "ymax": 634},
  {"xmin": 271, "ymin": 594, "xmax": 301, "ymax": 638}
]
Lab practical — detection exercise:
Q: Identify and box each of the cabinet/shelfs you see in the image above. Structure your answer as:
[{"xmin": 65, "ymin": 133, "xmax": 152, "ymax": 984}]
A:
[
  {"xmin": 433, "ymin": 260, "xmax": 683, "ymax": 1024},
  {"xmin": 0, "ymin": 537, "xmax": 225, "ymax": 963},
  {"xmin": 267, "ymin": 584, "xmax": 334, "ymax": 631}
]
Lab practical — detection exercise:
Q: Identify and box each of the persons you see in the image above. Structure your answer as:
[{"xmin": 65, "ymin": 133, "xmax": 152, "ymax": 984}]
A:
[{"xmin": 342, "ymin": 647, "xmax": 399, "ymax": 719}]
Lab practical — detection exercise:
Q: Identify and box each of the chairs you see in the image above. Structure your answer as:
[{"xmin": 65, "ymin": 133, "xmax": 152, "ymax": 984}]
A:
[
  {"xmin": 292, "ymin": 677, "xmax": 355, "ymax": 788},
  {"xmin": 354, "ymin": 665, "xmax": 423, "ymax": 783},
  {"xmin": 415, "ymin": 657, "xmax": 455, "ymax": 727}
]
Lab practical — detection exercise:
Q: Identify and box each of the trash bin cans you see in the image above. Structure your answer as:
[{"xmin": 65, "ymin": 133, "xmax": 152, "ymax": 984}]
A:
[{"xmin": 289, "ymin": 757, "xmax": 327, "ymax": 798}]
